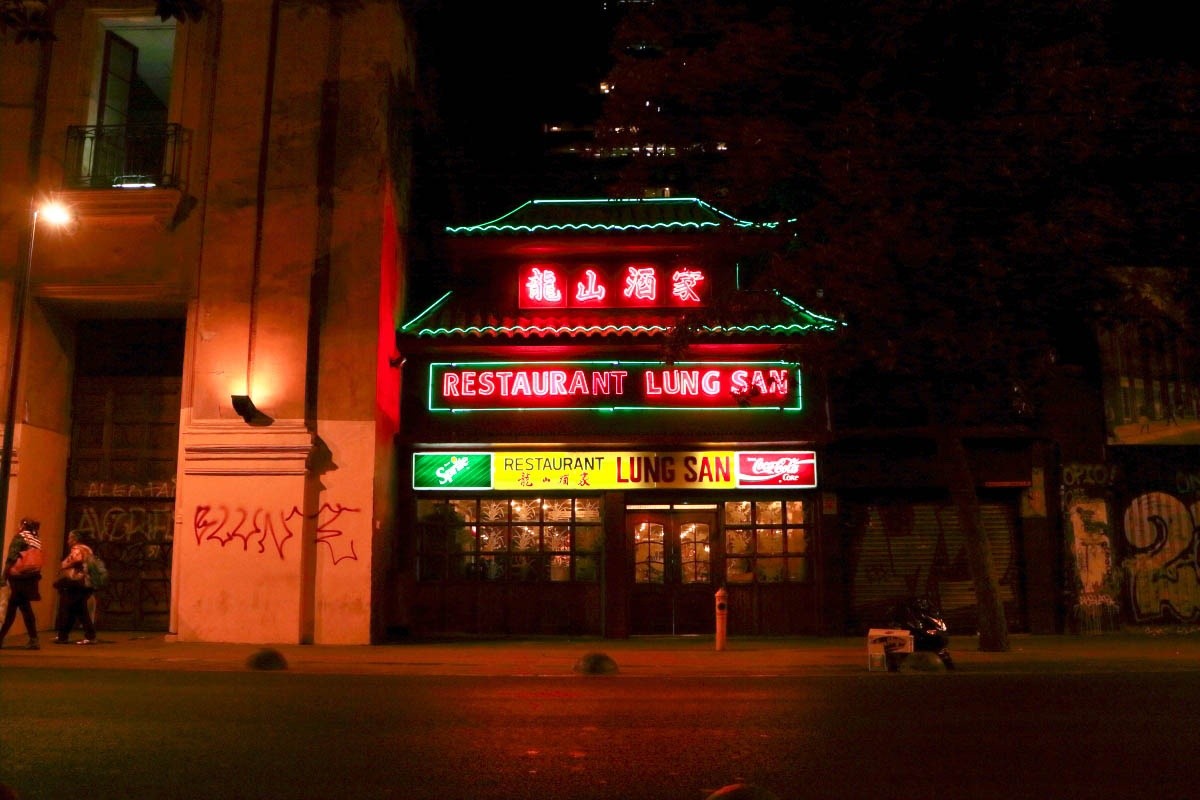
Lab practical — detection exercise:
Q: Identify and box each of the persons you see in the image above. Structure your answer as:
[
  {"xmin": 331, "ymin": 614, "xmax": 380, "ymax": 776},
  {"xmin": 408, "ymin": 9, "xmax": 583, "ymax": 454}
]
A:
[
  {"xmin": 48, "ymin": 530, "xmax": 98, "ymax": 644},
  {"xmin": 0, "ymin": 518, "xmax": 42, "ymax": 648}
]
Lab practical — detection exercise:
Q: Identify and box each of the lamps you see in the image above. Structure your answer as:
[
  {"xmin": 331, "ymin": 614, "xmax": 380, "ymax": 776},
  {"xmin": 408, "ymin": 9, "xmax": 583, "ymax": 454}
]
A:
[{"xmin": 229, "ymin": 393, "xmax": 260, "ymax": 420}]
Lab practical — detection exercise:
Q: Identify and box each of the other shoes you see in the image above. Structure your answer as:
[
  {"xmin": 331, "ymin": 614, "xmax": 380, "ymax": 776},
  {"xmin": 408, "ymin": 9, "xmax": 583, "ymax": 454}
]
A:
[
  {"xmin": 49, "ymin": 635, "xmax": 67, "ymax": 643},
  {"xmin": 23, "ymin": 638, "xmax": 40, "ymax": 647},
  {"xmin": 77, "ymin": 638, "xmax": 96, "ymax": 643}
]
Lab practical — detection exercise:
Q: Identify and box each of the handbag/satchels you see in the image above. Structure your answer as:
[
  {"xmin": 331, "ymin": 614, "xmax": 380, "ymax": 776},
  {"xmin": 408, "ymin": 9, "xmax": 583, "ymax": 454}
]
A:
[
  {"xmin": 79, "ymin": 545, "xmax": 109, "ymax": 589},
  {"xmin": 10, "ymin": 531, "xmax": 43, "ymax": 579},
  {"xmin": 54, "ymin": 546, "xmax": 85, "ymax": 589}
]
